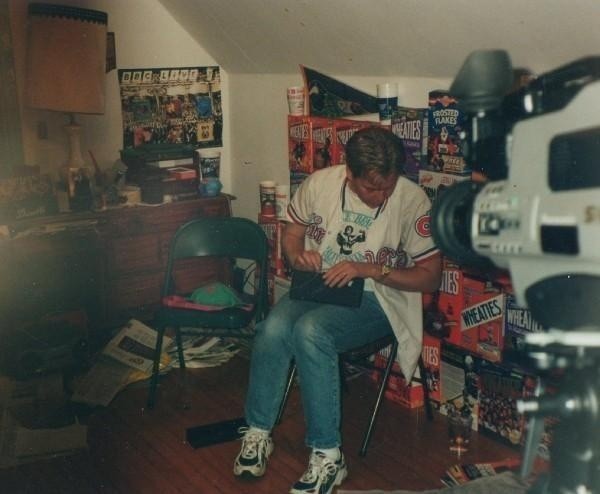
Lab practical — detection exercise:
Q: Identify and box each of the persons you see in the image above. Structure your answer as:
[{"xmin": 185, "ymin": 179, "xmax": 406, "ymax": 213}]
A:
[{"xmin": 233, "ymin": 126, "xmax": 444, "ymax": 494}]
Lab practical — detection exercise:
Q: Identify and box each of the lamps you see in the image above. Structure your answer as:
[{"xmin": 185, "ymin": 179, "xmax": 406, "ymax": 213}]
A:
[{"xmin": 21, "ymin": 0, "xmax": 108, "ymax": 179}]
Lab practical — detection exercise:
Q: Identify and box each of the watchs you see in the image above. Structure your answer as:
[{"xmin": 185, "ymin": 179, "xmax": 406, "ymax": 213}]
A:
[{"xmin": 381, "ymin": 265, "xmax": 393, "ymax": 284}]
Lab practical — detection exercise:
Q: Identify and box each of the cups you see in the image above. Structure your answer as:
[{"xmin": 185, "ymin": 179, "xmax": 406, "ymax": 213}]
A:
[
  {"xmin": 287, "ymin": 85, "xmax": 306, "ymax": 117},
  {"xmin": 258, "ymin": 181, "xmax": 291, "ymax": 220},
  {"xmin": 376, "ymin": 81, "xmax": 400, "ymax": 126},
  {"xmin": 447, "ymin": 410, "xmax": 474, "ymax": 455}
]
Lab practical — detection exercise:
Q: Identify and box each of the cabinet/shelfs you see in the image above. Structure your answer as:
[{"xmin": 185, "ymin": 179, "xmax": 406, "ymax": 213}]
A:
[
  {"xmin": 1, "ymin": 217, "xmax": 103, "ymax": 360},
  {"xmin": 98, "ymin": 198, "xmax": 232, "ymax": 331}
]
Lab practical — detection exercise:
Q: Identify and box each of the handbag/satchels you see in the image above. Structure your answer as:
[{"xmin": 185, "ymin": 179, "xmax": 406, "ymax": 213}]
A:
[{"xmin": 290, "ymin": 268, "xmax": 364, "ymax": 308}]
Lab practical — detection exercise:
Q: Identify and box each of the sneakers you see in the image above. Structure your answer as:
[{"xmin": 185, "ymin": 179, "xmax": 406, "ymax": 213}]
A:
[
  {"xmin": 234, "ymin": 426, "xmax": 274, "ymax": 479},
  {"xmin": 288, "ymin": 448, "xmax": 348, "ymax": 494}
]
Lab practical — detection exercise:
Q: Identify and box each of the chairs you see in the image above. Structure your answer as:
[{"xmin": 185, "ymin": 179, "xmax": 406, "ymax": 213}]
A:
[
  {"xmin": 145, "ymin": 217, "xmax": 271, "ymax": 411},
  {"xmin": 275, "ymin": 251, "xmax": 441, "ymax": 457}
]
